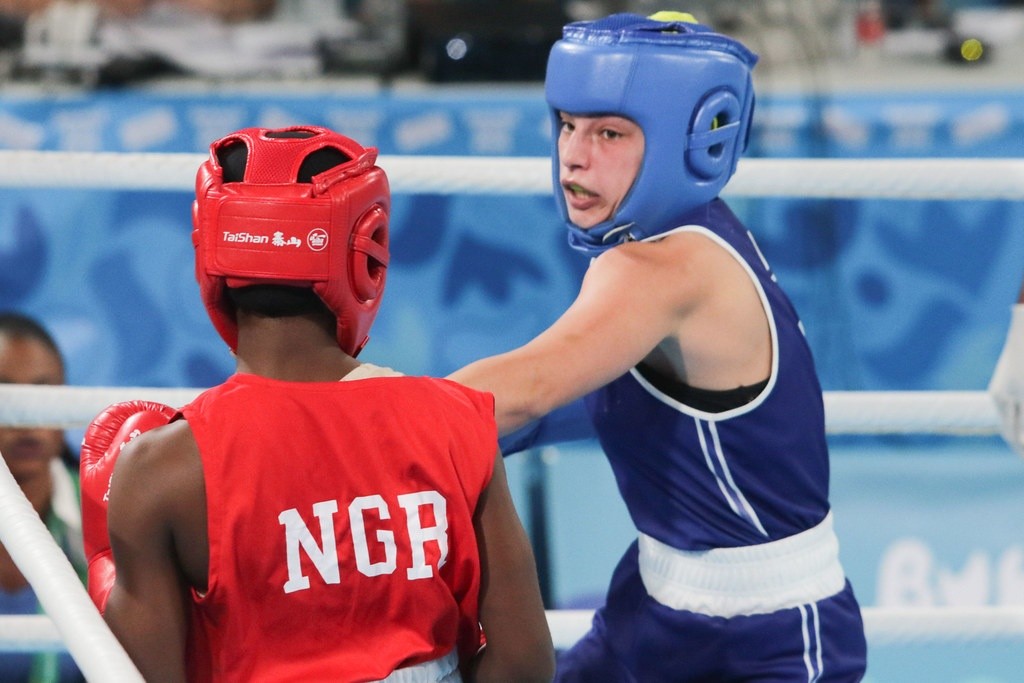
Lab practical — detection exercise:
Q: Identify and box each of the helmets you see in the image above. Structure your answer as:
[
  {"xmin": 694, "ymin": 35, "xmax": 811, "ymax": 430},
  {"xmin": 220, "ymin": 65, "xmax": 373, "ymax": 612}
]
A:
[
  {"xmin": 545, "ymin": 14, "xmax": 758, "ymax": 259},
  {"xmin": 190, "ymin": 125, "xmax": 391, "ymax": 356}
]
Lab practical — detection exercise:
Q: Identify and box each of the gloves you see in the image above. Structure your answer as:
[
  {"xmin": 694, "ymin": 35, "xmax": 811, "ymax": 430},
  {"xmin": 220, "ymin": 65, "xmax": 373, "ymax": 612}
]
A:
[{"xmin": 77, "ymin": 399, "xmax": 186, "ymax": 617}]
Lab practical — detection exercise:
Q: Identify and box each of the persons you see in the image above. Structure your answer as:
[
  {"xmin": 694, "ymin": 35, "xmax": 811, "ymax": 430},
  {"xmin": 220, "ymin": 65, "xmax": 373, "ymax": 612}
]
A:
[
  {"xmin": 0, "ymin": 310, "xmax": 90, "ymax": 682},
  {"xmin": 80, "ymin": 122, "xmax": 559, "ymax": 683},
  {"xmin": 437, "ymin": 9, "xmax": 869, "ymax": 683}
]
